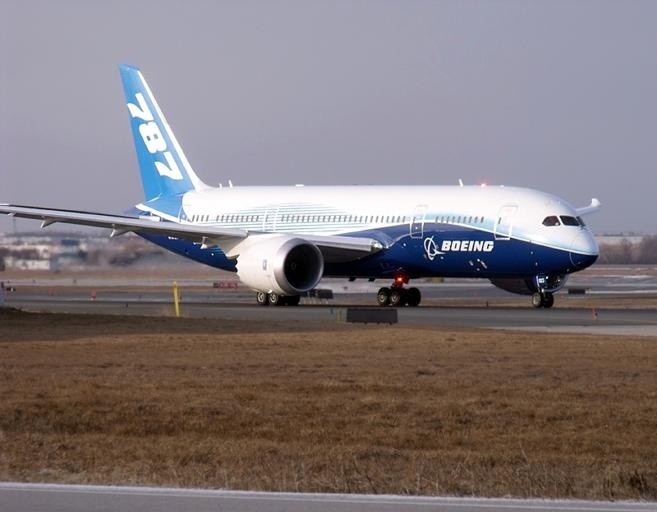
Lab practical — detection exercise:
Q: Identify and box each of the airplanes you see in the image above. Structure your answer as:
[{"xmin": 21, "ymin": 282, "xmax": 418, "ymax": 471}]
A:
[{"xmin": 0, "ymin": 58, "xmax": 605, "ymax": 307}]
